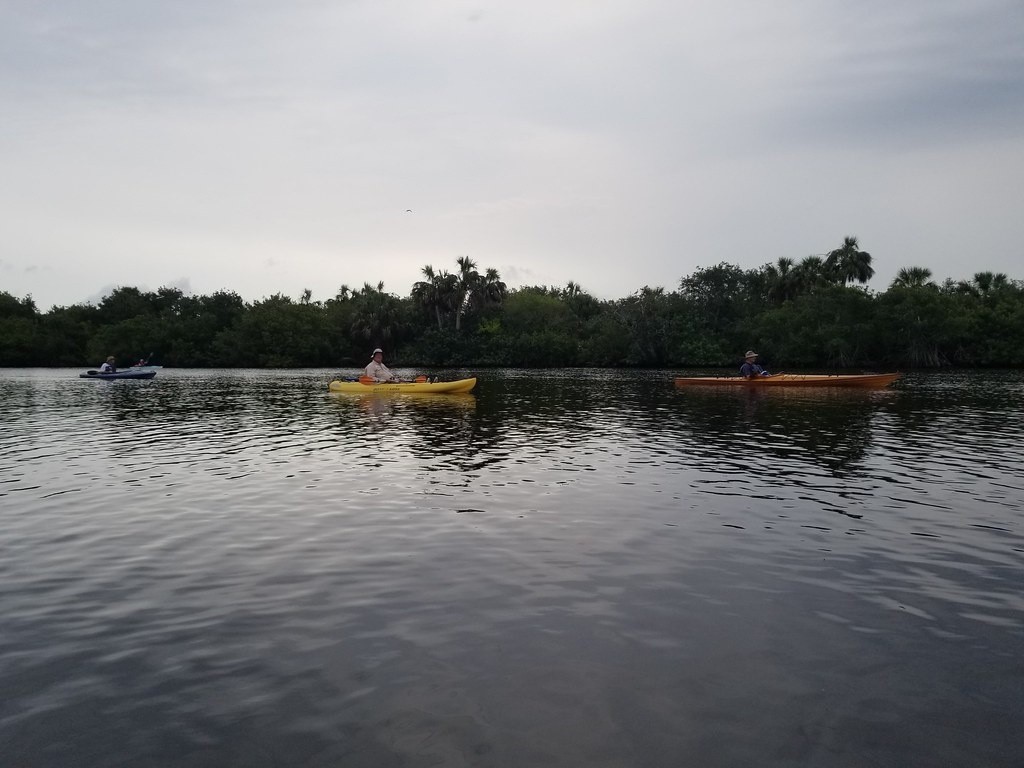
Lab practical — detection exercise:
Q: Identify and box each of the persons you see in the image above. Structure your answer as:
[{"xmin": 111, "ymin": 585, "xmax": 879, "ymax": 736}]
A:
[
  {"xmin": 100, "ymin": 356, "xmax": 117, "ymax": 373},
  {"xmin": 740, "ymin": 351, "xmax": 784, "ymax": 376},
  {"xmin": 364, "ymin": 348, "xmax": 438, "ymax": 383}
]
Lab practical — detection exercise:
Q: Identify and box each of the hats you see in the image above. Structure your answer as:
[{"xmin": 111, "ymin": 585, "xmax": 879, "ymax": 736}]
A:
[
  {"xmin": 106, "ymin": 356, "xmax": 116, "ymax": 362},
  {"xmin": 371, "ymin": 349, "xmax": 382, "ymax": 358},
  {"xmin": 744, "ymin": 351, "xmax": 758, "ymax": 357}
]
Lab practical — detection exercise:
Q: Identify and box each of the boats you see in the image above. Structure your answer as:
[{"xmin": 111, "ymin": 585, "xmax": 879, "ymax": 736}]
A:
[
  {"xmin": 80, "ymin": 369, "xmax": 156, "ymax": 381},
  {"xmin": 329, "ymin": 377, "xmax": 477, "ymax": 394},
  {"xmin": 130, "ymin": 366, "xmax": 163, "ymax": 369},
  {"xmin": 674, "ymin": 370, "xmax": 902, "ymax": 388}
]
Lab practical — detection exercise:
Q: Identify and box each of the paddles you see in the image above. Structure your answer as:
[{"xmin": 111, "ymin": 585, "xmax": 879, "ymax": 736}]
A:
[
  {"xmin": 748, "ymin": 370, "xmax": 785, "ymax": 378},
  {"xmin": 358, "ymin": 375, "xmax": 426, "ymax": 386},
  {"xmin": 86, "ymin": 370, "xmax": 128, "ymax": 375}
]
